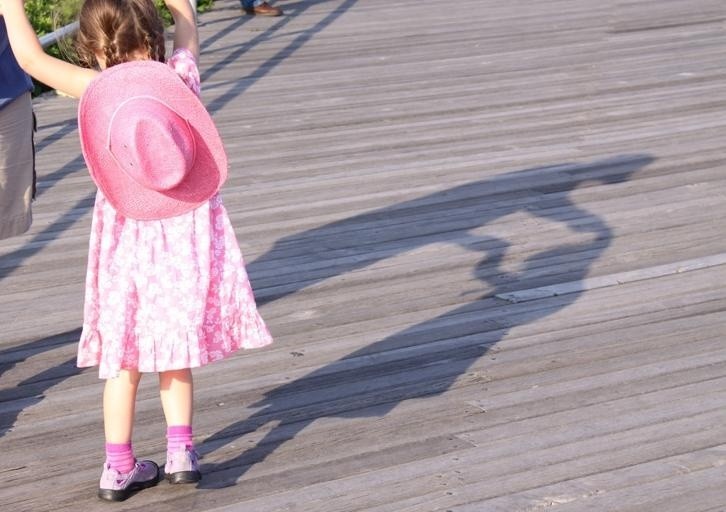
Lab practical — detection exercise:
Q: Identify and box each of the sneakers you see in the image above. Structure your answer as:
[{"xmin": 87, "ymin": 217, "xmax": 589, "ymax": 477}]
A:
[
  {"xmin": 98, "ymin": 460, "xmax": 159, "ymax": 501},
  {"xmin": 164, "ymin": 447, "xmax": 203, "ymax": 485}
]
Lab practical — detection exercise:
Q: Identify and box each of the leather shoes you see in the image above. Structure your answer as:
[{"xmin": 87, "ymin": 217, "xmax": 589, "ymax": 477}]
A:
[{"xmin": 245, "ymin": 2, "xmax": 283, "ymax": 16}]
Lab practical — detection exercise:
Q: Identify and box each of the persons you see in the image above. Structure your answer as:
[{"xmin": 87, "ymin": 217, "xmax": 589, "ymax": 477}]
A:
[
  {"xmin": 0, "ymin": 1, "xmax": 39, "ymax": 242},
  {"xmin": 237, "ymin": 0, "xmax": 287, "ymax": 18},
  {"xmin": 0, "ymin": 1, "xmax": 275, "ymax": 502}
]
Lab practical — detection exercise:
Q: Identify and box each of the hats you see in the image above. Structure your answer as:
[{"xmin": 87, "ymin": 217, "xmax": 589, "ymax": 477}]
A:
[{"xmin": 78, "ymin": 61, "xmax": 228, "ymax": 222}]
